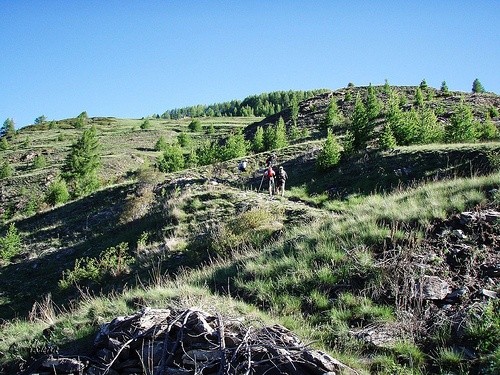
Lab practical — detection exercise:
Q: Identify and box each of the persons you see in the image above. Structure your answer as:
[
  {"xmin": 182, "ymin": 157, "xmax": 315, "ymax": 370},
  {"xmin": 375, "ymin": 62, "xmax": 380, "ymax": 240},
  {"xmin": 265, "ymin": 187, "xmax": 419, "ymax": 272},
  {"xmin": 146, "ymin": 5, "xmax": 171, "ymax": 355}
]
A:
[
  {"xmin": 264, "ymin": 166, "xmax": 276, "ymax": 196},
  {"xmin": 275, "ymin": 166, "xmax": 288, "ymax": 197}
]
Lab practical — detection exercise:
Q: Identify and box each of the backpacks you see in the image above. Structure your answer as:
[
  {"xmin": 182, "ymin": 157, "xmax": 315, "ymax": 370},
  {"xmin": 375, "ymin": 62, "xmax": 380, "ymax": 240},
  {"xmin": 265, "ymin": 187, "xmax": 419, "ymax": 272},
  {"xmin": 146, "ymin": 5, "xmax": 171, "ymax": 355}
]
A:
[
  {"xmin": 266, "ymin": 168, "xmax": 274, "ymax": 178},
  {"xmin": 278, "ymin": 169, "xmax": 285, "ymax": 179}
]
